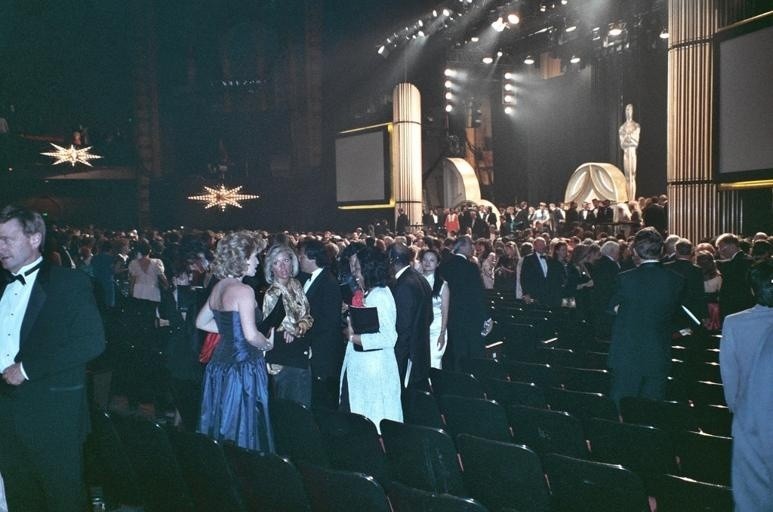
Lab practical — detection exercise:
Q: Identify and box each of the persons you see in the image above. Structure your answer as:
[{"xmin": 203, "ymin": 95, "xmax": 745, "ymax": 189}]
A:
[
  {"xmin": 3, "ymin": 193, "xmax": 772, "ymax": 511},
  {"xmin": 0, "ymin": 104, "xmax": 20, "ymax": 139},
  {"xmin": 618, "ymin": 101, "xmax": 641, "ymax": 200}
]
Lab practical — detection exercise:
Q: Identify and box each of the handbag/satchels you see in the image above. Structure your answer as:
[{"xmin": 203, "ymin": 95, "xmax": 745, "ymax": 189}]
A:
[{"xmin": 198, "ymin": 334, "xmax": 220, "ymax": 363}]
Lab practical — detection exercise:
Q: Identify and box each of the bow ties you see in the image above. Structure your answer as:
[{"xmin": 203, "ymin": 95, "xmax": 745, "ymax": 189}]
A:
[
  {"xmin": 5, "ymin": 274, "xmax": 27, "ymax": 285},
  {"xmin": 540, "ymin": 256, "xmax": 547, "ymax": 259}
]
